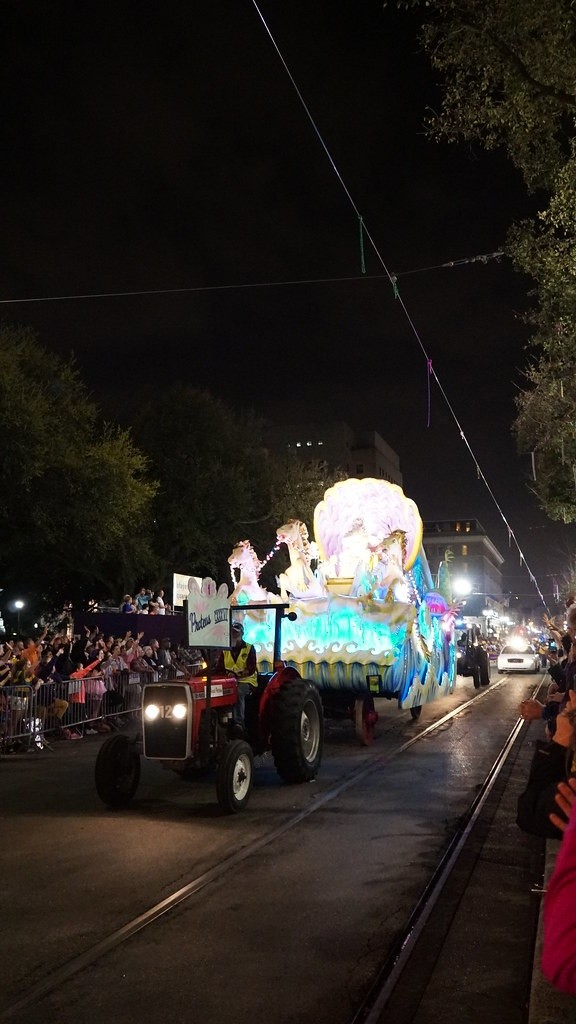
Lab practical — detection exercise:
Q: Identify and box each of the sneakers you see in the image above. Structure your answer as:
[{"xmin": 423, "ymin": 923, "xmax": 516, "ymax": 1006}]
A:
[
  {"xmin": 63, "ymin": 729, "xmax": 69, "ymax": 737},
  {"xmin": 86, "ymin": 728, "xmax": 98, "ymax": 735},
  {"xmin": 67, "ymin": 731, "xmax": 81, "ymax": 739}
]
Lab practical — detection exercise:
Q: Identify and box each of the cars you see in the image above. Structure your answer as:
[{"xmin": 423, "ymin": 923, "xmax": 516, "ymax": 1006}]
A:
[{"xmin": 497, "ymin": 644, "xmax": 542, "ymax": 675}]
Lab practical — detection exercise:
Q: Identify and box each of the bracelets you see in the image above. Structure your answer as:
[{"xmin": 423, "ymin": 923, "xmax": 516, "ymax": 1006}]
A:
[{"xmin": 547, "ymin": 624, "xmax": 552, "ymax": 629}]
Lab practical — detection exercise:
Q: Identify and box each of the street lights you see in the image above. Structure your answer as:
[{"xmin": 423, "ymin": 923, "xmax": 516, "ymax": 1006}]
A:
[{"xmin": 14, "ymin": 600, "xmax": 24, "ymax": 632}]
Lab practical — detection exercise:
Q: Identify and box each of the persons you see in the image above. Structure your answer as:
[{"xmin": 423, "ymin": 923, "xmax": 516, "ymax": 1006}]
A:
[
  {"xmin": 0, "ymin": 585, "xmax": 222, "ymax": 758},
  {"xmin": 221, "ymin": 623, "xmax": 259, "ymax": 733},
  {"xmin": 516, "ymin": 598, "xmax": 576, "ymax": 1024},
  {"xmin": 447, "ymin": 612, "xmax": 555, "ymax": 669}
]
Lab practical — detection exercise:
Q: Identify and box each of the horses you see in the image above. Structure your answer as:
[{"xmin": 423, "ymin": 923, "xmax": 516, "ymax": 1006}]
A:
[{"xmin": 227, "ymin": 519, "xmax": 422, "ymax": 604}]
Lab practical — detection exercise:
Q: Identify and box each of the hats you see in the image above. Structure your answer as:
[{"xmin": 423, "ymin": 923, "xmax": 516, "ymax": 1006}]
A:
[{"xmin": 232, "ymin": 623, "xmax": 244, "ymax": 633}]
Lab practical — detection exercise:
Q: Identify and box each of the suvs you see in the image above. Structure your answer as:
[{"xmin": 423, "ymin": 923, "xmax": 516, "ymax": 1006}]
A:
[{"xmin": 455, "ymin": 621, "xmax": 491, "ymax": 688}]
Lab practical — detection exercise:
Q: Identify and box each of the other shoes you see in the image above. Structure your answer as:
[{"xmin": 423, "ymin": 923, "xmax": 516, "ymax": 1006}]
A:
[
  {"xmin": 8, "ymin": 751, "xmax": 16, "ymax": 754},
  {"xmin": 236, "ymin": 722, "xmax": 242, "ymax": 738},
  {"xmin": 54, "ymin": 716, "xmax": 63, "ymax": 729},
  {"xmin": 114, "ymin": 716, "xmax": 125, "ymax": 725}
]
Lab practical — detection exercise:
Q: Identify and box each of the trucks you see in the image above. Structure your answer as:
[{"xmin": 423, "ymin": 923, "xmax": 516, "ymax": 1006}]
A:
[{"xmin": 94, "ymin": 602, "xmax": 424, "ymax": 814}]
